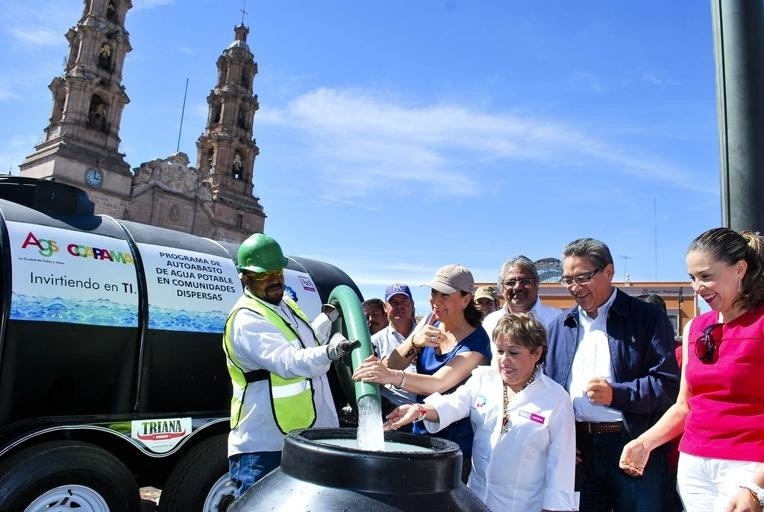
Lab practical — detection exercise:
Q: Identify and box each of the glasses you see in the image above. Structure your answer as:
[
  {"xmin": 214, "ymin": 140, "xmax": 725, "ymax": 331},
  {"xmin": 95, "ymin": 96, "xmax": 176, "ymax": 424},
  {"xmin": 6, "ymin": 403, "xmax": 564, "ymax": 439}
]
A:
[
  {"xmin": 559, "ymin": 270, "xmax": 598, "ymax": 285},
  {"xmin": 695, "ymin": 324, "xmax": 722, "ymax": 361}
]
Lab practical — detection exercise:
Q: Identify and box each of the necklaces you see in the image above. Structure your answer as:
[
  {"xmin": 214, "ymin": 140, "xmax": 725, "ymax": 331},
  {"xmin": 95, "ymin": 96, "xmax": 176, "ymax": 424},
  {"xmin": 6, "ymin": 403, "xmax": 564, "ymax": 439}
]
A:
[{"xmin": 502, "ymin": 365, "xmax": 539, "ymax": 432}]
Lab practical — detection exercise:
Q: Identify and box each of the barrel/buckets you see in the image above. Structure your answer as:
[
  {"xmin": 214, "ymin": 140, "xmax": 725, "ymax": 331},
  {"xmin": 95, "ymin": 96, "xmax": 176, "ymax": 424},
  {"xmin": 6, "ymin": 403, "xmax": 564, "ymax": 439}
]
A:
[{"xmin": 224, "ymin": 426, "xmax": 495, "ymax": 512}]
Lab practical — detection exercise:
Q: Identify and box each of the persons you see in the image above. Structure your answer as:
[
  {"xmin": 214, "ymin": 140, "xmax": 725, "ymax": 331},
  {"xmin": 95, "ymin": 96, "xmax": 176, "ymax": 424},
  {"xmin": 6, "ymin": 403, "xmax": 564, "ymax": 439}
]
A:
[
  {"xmin": 633, "ymin": 294, "xmax": 682, "ymax": 373},
  {"xmin": 379, "ymin": 311, "xmax": 578, "ymax": 512},
  {"xmin": 619, "ymin": 226, "xmax": 764, "ymax": 512},
  {"xmin": 479, "ymin": 254, "xmax": 564, "ymax": 369},
  {"xmin": 221, "ymin": 232, "xmax": 348, "ymax": 502},
  {"xmin": 542, "ymin": 236, "xmax": 685, "ymax": 512},
  {"xmin": 369, "ymin": 284, "xmax": 419, "ymax": 406},
  {"xmin": 472, "ymin": 286, "xmax": 500, "ymax": 321},
  {"xmin": 350, "ymin": 263, "xmax": 491, "ymax": 485},
  {"xmin": 359, "ymin": 297, "xmax": 388, "ymax": 336}
]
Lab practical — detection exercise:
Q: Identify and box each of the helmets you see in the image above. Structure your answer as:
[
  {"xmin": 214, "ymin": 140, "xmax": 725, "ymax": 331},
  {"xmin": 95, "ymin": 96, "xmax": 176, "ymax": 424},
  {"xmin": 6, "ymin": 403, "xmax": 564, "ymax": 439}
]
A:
[{"xmin": 238, "ymin": 233, "xmax": 288, "ymax": 273}]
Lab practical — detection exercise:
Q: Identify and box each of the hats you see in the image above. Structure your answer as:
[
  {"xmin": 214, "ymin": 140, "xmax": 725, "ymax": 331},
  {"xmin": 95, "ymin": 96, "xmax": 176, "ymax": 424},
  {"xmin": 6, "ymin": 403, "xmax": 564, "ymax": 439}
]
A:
[
  {"xmin": 420, "ymin": 265, "xmax": 474, "ymax": 294},
  {"xmin": 474, "ymin": 287, "xmax": 498, "ymax": 303},
  {"xmin": 386, "ymin": 284, "xmax": 412, "ymax": 302}
]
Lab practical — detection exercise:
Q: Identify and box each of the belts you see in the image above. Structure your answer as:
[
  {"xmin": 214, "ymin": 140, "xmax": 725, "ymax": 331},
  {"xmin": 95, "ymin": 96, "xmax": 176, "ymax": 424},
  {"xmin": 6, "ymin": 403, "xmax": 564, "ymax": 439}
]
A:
[{"xmin": 578, "ymin": 423, "xmax": 621, "ymax": 434}]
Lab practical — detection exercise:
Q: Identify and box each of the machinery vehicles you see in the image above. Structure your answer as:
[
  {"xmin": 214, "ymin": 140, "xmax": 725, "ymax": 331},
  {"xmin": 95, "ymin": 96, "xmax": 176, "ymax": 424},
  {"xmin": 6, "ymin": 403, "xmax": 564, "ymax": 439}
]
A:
[{"xmin": 3, "ymin": 170, "xmax": 366, "ymax": 509}]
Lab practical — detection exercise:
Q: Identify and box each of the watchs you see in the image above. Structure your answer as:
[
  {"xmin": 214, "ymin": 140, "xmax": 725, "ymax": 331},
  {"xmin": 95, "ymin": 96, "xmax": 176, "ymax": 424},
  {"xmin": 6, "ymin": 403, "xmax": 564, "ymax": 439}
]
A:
[{"xmin": 739, "ymin": 480, "xmax": 764, "ymax": 507}]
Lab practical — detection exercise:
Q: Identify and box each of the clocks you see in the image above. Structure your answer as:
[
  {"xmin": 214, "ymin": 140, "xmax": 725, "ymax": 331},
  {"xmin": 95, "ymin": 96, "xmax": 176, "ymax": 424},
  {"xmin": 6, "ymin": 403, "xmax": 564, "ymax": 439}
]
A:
[{"xmin": 84, "ymin": 166, "xmax": 105, "ymax": 189}]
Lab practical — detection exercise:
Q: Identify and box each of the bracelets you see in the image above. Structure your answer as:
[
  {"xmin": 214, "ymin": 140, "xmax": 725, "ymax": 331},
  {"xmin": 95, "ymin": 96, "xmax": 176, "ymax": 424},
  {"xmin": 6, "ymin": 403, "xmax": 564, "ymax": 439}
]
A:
[
  {"xmin": 411, "ymin": 401, "xmax": 427, "ymax": 423},
  {"xmin": 411, "ymin": 334, "xmax": 419, "ymax": 350},
  {"xmin": 393, "ymin": 369, "xmax": 407, "ymax": 389}
]
